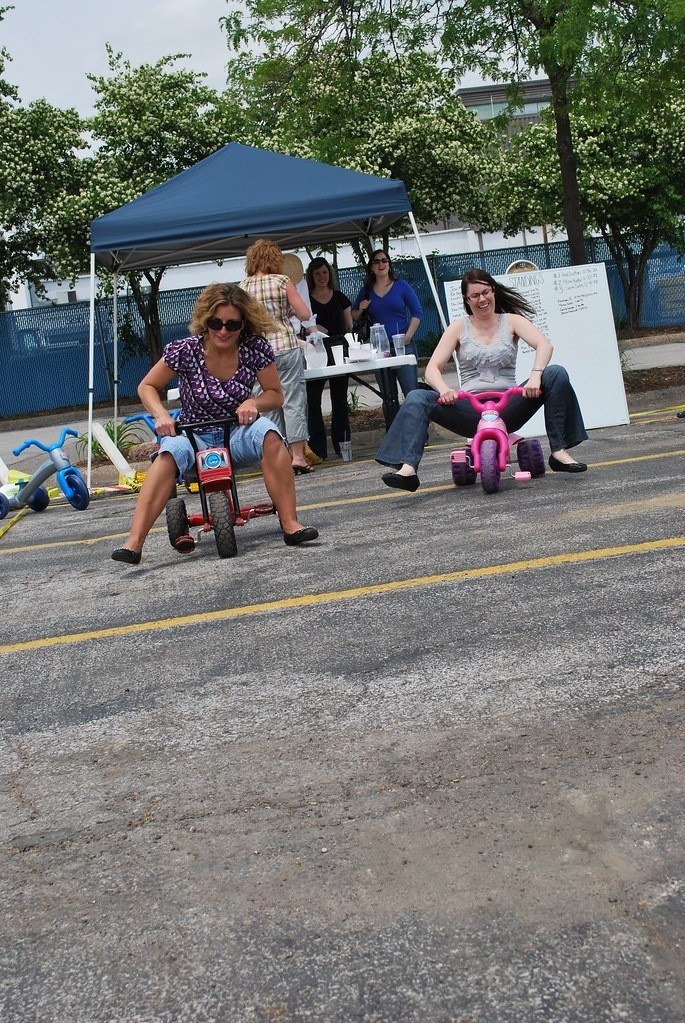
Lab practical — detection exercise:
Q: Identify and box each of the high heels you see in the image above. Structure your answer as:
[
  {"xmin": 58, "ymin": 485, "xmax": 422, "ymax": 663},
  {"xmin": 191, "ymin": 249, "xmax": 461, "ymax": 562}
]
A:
[{"xmin": 293, "ymin": 465, "xmax": 315, "ymax": 475}]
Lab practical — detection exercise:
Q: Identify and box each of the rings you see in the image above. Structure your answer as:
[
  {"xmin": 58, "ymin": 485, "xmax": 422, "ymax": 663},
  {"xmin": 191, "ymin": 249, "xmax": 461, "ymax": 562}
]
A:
[{"xmin": 248, "ymin": 417, "xmax": 252, "ymax": 420}]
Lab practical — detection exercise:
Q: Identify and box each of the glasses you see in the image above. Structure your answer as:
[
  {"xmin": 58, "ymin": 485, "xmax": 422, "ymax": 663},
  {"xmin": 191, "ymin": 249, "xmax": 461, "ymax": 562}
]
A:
[
  {"xmin": 466, "ymin": 288, "xmax": 493, "ymax": 301},
  {"xmin": 371, "ymin": 258, "xmax": 389, "ymax": 264},
  {"xmin": 206, "ymin": 316, "xmax": 247, "ymax": 332}
]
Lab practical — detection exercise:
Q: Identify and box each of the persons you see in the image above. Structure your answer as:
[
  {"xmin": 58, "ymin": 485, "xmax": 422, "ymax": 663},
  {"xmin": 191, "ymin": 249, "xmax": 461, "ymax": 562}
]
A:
[
  {"xmin": 374, "ymin": 268, "xmax": 588, "ymax": 492},
  {"xmin": 351, "ymin": 250, "xmax": 429, "ymax": 447},
  {"xmin": 236, "ymin": 240, "xmax": 315, "ymax": 474},
  {"xmin": 287, "ymin": 279, "xmax": 322, "ymax": 463},
  {"xmin": 301, "ymin": 257, "xmax": 354, "ymax": 461},
  {"xmin": 111, "ymin": 282, "xmax": 319, "ymax": 565}
]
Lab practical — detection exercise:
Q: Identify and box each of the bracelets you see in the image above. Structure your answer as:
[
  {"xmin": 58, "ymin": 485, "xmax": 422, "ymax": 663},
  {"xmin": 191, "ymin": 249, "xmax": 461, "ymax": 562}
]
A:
[{"xmin": 531, "ymin": 368, "xmax": 545, "ymax": 371}]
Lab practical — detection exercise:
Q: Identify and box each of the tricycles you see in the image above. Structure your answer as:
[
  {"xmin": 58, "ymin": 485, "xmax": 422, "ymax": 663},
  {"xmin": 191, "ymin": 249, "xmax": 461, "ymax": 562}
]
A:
[
  {"xmin": 438, "ymin": 386, "xmax": 546, "ymax": 495},
  {"xmin": 123, "ymin": 408, "xmax": 199, "ymax": 494},
  {"xmin": 154, "ymin": 411, "xmax": 282, "ymax": 560},
  {"xmin": 0, "ymin": 427, "xmax": 91, "ymax": 520}
]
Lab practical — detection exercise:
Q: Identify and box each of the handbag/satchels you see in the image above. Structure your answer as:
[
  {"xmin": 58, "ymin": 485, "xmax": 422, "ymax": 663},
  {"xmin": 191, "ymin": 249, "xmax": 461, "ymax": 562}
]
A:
[{"xmin": 352, "ymin": 283, "xmax": 372, "ymax": 344}]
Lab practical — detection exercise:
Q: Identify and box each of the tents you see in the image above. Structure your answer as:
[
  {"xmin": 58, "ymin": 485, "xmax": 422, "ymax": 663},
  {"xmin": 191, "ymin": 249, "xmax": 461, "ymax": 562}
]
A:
[{"xmin": 85, "ymin": 141, "xmax": 461, "ymax": 491}]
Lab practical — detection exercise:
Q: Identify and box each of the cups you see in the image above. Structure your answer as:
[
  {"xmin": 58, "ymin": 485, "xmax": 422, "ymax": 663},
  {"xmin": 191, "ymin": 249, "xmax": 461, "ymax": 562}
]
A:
[
  {"xmin": 339, "ymin": 441, "xmax": 351, "ymax": 461},
  {"xmin": 348, "ymin": 343, "xmax": 370, "ymax": 359},
  {"xmin": 392, "ymin": 333, "xmax": 406, "ymax": 356},
  {"xmin": 331, "ymin": 345, "xmax": 344, "ymax": 366}
]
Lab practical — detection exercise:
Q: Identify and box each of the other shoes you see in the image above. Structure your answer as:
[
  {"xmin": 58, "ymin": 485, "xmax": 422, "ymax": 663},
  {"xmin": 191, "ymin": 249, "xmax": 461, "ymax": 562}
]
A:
[
  {"xmin": 382, "ymin": 473, "xmax": 420, "ymax": 492},
  {"xmin": 303, "ymin": 450, "xmax": 323, "ymax": 467},
  {"xmin": 283, "ymin": 526, "xmax": 319, "ymax": 546},
  {"xmin": 677, "ymin": 411, "xmax": 685, "ymax": 418},
  {"xmin": 548, "ymin": 454, "xmax": 587, "ymax": 473},
  {"xmin": 111, "ymin": 548, "xmax": 141, "ymax": 564}
]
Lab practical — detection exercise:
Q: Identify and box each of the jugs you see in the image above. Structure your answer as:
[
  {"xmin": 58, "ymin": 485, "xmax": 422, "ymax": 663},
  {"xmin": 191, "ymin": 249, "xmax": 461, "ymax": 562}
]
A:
[
  {"xmin": 305, "ymin": 334, "xmax": 328, "ymax": 369},
  {"xmin": 370, "ymin": 322, "xmax": 390, "ymax": 357}
]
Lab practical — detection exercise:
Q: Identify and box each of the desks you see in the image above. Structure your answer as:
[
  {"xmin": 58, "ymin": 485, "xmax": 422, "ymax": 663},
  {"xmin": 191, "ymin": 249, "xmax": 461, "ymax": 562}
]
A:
[{"xmin": 303, "ymin": 354, "xmax": 417, "ymax": 434}]
